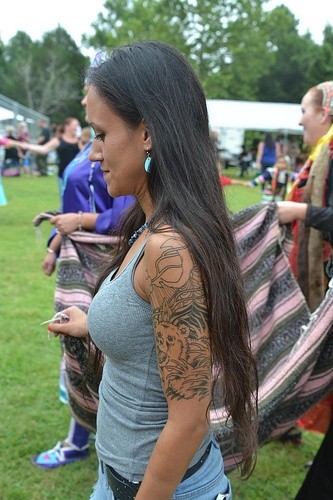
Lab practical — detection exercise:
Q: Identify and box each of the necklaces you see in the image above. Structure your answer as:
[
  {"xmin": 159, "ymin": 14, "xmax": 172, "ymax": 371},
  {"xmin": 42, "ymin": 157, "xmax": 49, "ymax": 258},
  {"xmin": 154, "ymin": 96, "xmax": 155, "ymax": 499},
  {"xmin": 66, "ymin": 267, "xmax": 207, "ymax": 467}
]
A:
[{"xmin": 127, "ymin": 221, "xmax": 149, "ymax": 246}]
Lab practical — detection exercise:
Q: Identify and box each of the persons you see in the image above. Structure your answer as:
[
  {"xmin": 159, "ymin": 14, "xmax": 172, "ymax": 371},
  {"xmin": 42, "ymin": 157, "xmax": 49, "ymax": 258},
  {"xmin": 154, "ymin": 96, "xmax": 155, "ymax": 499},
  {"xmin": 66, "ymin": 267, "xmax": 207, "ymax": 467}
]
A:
[
  {"xmin": 268, "ymin": 78, "xmax": 333, "ymax": 446},
  {"xmin": 33, "ymin": 70, "xmax": 137, "ymax": 470},
  {"xmin": 204, "ymin": 132, "xmax": 310, "ymax": 202},
  {"xmin": 275, "ymin": 150, "xmax": 333, "ymax": 500},
  {"xmin": 48, "ymin": 40, "xmax": 257, "ymax": 500},
  {"xmin": 0, "ymin": 117, "xmax": 99, "ymax": 205}
]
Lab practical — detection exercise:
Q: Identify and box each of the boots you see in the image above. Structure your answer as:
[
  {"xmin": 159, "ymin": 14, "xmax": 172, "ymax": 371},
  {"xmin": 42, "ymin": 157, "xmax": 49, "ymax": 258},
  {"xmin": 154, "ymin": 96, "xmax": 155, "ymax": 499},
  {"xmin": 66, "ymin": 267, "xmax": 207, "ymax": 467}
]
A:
[{"xmin": 36, "ymin": 417, "xmax": 93, "ymax": 468}]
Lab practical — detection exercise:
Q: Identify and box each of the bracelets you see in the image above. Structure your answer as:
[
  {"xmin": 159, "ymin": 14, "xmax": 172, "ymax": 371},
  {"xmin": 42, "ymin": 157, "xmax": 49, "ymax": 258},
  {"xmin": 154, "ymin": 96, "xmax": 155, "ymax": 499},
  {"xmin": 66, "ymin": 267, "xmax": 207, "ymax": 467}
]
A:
[
  {"xmin": 78, "ymin": 211, "xmax": 82, "ymax": 231},
  {"xmin": 47, "ymin": 247, "xmax": 54, "ymax": 253}
]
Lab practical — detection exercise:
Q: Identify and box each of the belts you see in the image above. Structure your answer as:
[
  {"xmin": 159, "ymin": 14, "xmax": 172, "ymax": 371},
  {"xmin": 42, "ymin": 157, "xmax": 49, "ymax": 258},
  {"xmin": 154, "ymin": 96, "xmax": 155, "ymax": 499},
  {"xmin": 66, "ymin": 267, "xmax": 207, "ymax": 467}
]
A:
[{"xmin": 104, "ymin": 440, "xmax": 211, "ymax": 500}]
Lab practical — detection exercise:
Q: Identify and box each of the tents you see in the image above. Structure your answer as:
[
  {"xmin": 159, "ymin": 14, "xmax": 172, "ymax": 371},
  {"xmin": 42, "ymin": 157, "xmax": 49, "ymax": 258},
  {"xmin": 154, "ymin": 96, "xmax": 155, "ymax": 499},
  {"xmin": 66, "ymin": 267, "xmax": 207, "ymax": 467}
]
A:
[{"xmin": 204, "ymin": 99, "xmax": 304, "ymax": 165}]
